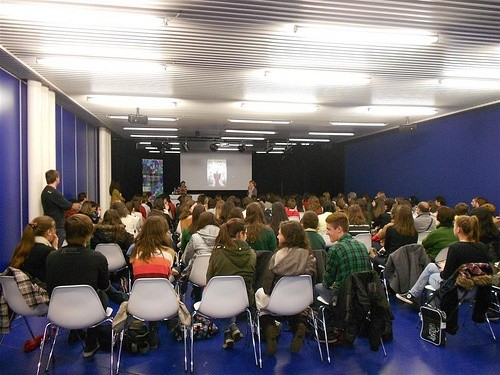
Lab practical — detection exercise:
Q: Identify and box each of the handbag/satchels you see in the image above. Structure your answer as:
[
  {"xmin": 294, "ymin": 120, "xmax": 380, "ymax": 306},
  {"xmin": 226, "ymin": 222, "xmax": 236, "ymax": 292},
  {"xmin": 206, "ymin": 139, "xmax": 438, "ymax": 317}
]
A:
[
  {"xmin": 419, "ymin": 302, "xmax": 446, "ymax": 346},
  {"xmin": 121, "ymin": 320, "xmax": 150, "ymax": 354},
  {"xmin": 173, "ymin": 314, "xmax": 219, "ymax": 344}
]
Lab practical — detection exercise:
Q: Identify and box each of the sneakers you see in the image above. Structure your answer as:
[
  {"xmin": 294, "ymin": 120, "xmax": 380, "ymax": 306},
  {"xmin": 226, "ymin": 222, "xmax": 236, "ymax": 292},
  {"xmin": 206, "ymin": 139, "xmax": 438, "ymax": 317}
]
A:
[{"xmin": 395, "ymin": 291, "xmax": 415, "ymax": 305}]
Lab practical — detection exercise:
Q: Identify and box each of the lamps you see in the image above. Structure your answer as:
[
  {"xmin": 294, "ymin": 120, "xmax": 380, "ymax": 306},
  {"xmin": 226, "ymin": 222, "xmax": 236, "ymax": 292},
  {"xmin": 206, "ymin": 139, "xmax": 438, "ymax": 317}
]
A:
[
  {"xmin": 207, "ymin": 139, "xmax": 219, "ymax": 152},
  {"xmin": 266, "ymin": 138, "xmax": 273, "ymax": 154},
  {"xmin": 180, "ymin": 139, "xmax": 190, "ymax": 153},
  {"xmin": 238, "ymin": 139, "xmax": 247, "ymax": 153}
]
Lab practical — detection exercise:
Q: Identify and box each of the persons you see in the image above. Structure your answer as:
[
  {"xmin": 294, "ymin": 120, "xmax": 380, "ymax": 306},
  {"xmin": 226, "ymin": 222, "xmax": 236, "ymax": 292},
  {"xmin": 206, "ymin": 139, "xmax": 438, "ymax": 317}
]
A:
[
  {"xmin": 177, "ymin": 181, "xmax": 187, "ymax": 194},
  {"xmin": 268, "ymin": 192, "xmax": 500, "ymax": 274},
  {"xmin": 260, "ymin": 221, "xmax": 317, "ymax": 357},
  {"xmin": 47, "ymin": 214, "xmax": 131, "ymax": 357},
  {"xmin": 65, "ymin": 192, "xmax": 326, "ymax": 302},
  {"xmin": 10, "ymin": 216, "xmax": 84, "ymax": 346},
  {"xmin": 110, "ymin": 179, "xmax": 125, "ymax": 206},
  {"xmin": 207, "ymin": 218, "xmax": 257, "ymax": 347},
  {"xmin": 129, "ymin": 216, "xmax": 176, "ymax": 281},
  {"xmin": 248, "ymin": 181, "xmax": 257, "ymax": 198},
  {"xmin": 41, "ymin": 170, "xmax": 72, "ymax": 249},
  {"xmin": 314, "ymin": 212, "xmax": 371, "ymax": 342},
  {"xmin": 396, "ymin": 216, "xmax": 485, "ymax": 304}
]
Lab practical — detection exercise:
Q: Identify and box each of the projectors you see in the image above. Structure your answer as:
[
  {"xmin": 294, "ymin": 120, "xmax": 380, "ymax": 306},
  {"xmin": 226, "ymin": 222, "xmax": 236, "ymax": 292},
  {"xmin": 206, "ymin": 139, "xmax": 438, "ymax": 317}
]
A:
[{"xmin": 127, "ymin": 114, "xmax": 148, "ymax": 124}]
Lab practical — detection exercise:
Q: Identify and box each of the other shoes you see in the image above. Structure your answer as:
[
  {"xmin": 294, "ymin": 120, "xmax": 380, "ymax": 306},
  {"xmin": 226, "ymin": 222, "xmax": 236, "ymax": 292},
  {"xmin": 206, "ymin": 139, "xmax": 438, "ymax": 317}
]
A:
[
  {"xmin": 83, "ymin": 340, "xmax": 100, "ymax": 358},
  {"xmin": 290, "ymin": 323, "xmax": 306, "ymax": 352},
  {"xmin": 222, "ymin": 332, "xmax": 234, "ymax": 349},
  {"xmin": 230, "ymin": 324, "xmax": 242, "ymax": 341},
  {"xmin": 67, "ymin": 335, "xmax": 81, "ymax": 346},
  {"xmin": 266, "ymin": 325, "xmax": 277, "ymax": 357},
  {"xmin": 313, "ymin": 332, "xmax": 337, "ymax": 342}
]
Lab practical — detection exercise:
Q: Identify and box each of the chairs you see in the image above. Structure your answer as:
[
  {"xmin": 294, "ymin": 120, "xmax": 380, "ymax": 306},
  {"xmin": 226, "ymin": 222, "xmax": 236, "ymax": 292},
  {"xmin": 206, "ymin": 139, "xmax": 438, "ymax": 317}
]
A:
[{"xmin": 0, "ymin": 232, "xmax": 500, "ymax": 375}]
